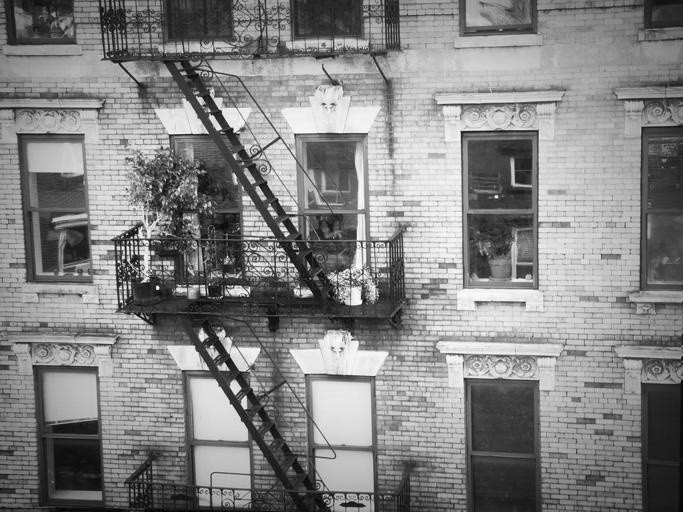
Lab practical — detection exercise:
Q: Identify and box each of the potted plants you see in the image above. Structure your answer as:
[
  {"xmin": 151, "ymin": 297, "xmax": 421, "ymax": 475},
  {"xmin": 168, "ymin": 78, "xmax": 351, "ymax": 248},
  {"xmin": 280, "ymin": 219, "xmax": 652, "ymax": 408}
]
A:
[
  {"xmin": 471, "ymin": 217, "xmax": 518, "ymax": 281},
  {"xmin": 125, "ymin": 146, "xmax": 217, "ymax": 306}
]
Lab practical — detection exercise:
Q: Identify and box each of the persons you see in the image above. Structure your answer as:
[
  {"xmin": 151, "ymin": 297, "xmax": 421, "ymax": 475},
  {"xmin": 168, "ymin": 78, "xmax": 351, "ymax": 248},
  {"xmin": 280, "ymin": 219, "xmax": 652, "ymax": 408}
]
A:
[
  {"xmin": 47, "ymin": 213, "xmax": 84, "ymax": 272},
  {"xmin": 310, "ymin": 219, "xmax": 352, "ymax": 255}
]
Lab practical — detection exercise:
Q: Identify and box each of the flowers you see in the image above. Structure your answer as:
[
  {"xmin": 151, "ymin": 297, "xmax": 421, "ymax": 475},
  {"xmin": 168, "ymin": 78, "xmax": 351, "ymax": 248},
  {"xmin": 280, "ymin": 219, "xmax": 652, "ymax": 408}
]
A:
[{"xmin": 327, "ymin": 267, "xmax": 381, "ymax": 305}]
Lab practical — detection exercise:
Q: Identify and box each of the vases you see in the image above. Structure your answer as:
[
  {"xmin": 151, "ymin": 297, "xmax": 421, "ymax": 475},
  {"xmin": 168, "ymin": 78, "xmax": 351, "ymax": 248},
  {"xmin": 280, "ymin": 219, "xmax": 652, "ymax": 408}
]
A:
[{"xmin": 340, "ymin": 286, "xmax": 363, "ymax": 306}]
